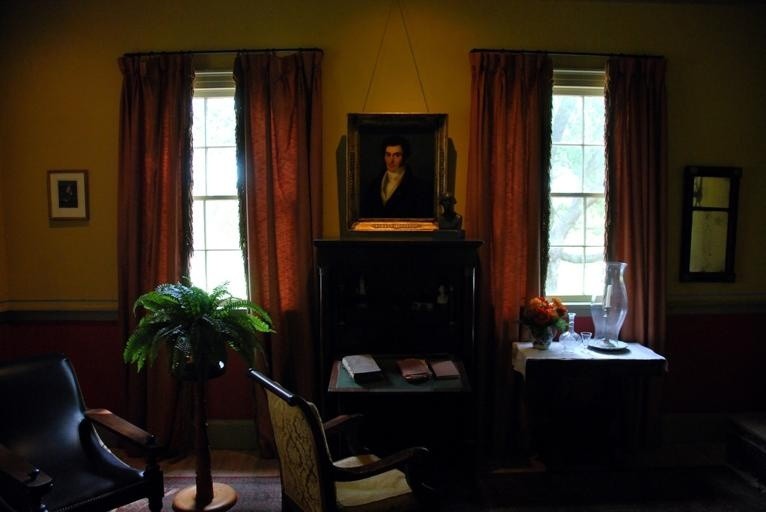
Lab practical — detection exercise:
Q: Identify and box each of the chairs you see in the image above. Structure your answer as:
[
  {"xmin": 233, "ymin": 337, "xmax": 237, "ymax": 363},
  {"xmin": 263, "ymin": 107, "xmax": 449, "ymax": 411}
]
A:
[
  {"xmin": 0, "ymin": 351, "xmax": 165, "ymax": 512},
  {"xmin": 247, "ymin": 361, "xmax": 435, "ymax": 512}
]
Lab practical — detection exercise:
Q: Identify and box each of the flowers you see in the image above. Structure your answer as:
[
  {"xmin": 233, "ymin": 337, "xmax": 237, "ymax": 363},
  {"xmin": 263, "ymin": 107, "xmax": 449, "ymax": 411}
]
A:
[{"xmin": 520, "ymin": 293, "xmax": 570, "ymax": 337}]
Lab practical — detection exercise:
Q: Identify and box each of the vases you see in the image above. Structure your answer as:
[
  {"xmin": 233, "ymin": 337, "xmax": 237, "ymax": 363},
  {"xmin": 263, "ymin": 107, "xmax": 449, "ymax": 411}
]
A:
[{"xmin": 532, "ymin": 327, "xmax": 555, "ymax": 351}]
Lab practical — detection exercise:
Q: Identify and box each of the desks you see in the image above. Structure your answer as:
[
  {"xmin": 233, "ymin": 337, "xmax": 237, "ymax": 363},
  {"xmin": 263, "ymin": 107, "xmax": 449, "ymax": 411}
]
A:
[
  {"xmin": 507, "ymin": 341, "xmax": 669, "ymax": 487},
  {"xmin": 323, "ymin": 355, "xmax": 493, "ymax": 508}
]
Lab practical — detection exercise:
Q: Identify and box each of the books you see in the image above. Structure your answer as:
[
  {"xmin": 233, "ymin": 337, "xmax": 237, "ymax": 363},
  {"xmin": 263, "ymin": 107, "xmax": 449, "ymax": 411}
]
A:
[
  {"xmin": 430, "ymin": 359, "xmax": 460, "ymax": 379},
  {"xmin": 341, "ymin": 353, "xmax": 383, "ymax": 383},
  {"xmin": 396, "ymin": 358, "xmax": 432, "ymax": 381}
]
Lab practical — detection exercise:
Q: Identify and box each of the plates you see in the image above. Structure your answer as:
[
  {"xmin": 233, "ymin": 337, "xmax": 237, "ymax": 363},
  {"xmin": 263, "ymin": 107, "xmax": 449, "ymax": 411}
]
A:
[{"xmin": 588, "ymin": 338, "xmax": 630, "ymax": 352}]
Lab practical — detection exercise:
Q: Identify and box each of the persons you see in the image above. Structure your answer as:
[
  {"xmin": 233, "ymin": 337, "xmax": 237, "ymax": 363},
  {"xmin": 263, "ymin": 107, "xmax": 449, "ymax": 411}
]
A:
[{"xmin": 367, "ymin": 138, "xmax": 427, "ymax": 218}]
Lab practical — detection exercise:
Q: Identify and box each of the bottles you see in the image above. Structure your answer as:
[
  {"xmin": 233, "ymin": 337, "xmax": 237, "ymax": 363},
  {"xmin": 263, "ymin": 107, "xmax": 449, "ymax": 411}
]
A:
[
  {"xmin": 588, "ymin": 261, "xmax": 630, "ymax": 347},
  {"xmin": 559, "ymin": 312, "xmax": 582, "ymax": 353}
]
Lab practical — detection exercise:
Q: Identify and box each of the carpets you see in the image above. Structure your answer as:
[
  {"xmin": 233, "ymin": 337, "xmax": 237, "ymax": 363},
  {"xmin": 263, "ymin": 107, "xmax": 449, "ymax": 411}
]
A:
[{"xmin": 485, "ymin": 461, "xmax": 766, "ymax": 511}]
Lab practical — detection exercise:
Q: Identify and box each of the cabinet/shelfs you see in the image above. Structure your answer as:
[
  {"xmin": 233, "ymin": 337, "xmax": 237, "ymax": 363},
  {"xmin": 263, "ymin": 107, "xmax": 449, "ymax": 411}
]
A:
[{"xmin": 309, "ymin": 232, "xmax": 484, "ymax": 445}]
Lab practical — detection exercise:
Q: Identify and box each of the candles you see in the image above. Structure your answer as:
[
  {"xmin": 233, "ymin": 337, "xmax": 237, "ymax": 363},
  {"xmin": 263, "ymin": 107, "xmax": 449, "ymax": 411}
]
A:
[{"xmin": 603, "ymin": 282, "xmax": 613, "ymax": 307}]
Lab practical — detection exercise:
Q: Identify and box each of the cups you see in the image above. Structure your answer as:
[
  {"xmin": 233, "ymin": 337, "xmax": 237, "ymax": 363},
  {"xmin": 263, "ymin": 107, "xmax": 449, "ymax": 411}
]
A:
[{"xmin": 580, "ymin": 332, "xmax": 593, "ymax": 350}]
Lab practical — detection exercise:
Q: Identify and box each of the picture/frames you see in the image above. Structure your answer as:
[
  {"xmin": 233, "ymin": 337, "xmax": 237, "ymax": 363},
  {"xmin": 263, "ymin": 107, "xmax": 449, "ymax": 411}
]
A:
[
  {"xmin": 43, "ymin": 166, "xmax": 90, "ymax": 222},
  {"xmin": 342, "ymin": 110, "xmax": 449, "ymax": 236}
]
